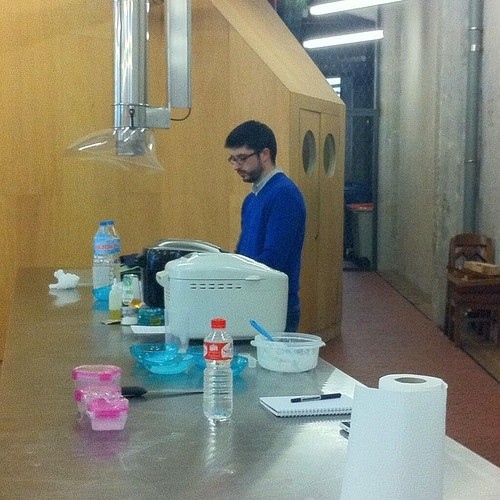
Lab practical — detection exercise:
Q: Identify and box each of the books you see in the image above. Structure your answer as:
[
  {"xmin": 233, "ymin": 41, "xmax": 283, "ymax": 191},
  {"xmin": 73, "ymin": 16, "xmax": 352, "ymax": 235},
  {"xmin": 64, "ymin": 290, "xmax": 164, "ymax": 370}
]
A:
[{"xmin": 259, "ymin": 395, "xmax": 353, "ymax": 418}]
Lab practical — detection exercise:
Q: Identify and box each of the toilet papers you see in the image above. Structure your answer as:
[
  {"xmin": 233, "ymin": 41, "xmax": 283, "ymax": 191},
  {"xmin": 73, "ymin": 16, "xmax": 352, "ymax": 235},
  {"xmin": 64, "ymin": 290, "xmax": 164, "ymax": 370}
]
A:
[{"xmin": 339, "ymin": 373, "xmax": 448, "ymax": 500}]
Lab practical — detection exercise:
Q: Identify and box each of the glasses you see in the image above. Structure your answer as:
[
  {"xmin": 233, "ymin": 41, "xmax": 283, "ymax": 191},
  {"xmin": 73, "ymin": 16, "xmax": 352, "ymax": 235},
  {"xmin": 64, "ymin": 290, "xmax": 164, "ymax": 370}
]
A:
[{"xmin": 228, "ymin": 147, "xmax": 268, "ymax": 164}]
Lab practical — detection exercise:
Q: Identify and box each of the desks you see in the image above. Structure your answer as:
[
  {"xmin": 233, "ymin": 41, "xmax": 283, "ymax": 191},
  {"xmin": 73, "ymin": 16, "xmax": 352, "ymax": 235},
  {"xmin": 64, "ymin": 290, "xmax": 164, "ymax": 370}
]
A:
[{"xmin": 0, "ymin": 265, "xmax": 500, "ymax": 500}]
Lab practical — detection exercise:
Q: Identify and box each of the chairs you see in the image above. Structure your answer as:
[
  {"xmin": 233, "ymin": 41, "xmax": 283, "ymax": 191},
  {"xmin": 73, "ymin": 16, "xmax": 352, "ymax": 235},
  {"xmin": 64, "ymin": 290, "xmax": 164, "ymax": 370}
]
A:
[{"xmin": 445, "ymin": 233, "xmax": 500, "ymax": 348}]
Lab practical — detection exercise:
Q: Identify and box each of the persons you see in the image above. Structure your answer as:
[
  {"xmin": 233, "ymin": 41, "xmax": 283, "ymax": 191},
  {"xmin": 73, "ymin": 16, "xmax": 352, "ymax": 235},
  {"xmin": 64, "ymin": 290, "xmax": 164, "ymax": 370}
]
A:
[{"xmin": 224, "ymin": 120, "xmax": 307, "ymax": 333}]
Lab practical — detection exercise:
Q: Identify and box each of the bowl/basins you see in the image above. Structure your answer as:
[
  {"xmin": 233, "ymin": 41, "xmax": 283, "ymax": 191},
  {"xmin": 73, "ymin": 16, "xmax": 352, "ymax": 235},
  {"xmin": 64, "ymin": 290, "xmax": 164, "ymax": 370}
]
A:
[
  {"xmin": 186, "ymin": 345, "xmax": 248, "ymax": 376},
  {"xmin": 250, "ymin": 332, "xmax": 326, "ymax": 373},
  {"xmin": 130, "ymin": 344, "xmax": 179, "ymax": 362},
  {"xmin": 136, "ymin": 306, "xmax": 164, "ymax": 326},
  {"xmin": 142, "ymin": 352, "xmax": 193, "ymax": 375},
  {"xmin": 72, "ymin": 365, "xmax": 128, "ymax": 433}
]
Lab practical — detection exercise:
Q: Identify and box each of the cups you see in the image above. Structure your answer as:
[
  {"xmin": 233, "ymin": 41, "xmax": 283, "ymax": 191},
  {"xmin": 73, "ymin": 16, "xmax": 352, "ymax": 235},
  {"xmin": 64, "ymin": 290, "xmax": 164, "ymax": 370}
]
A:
[{"xmin": 123, "ymin": 274, "xmax": 141, "ymax": 308}]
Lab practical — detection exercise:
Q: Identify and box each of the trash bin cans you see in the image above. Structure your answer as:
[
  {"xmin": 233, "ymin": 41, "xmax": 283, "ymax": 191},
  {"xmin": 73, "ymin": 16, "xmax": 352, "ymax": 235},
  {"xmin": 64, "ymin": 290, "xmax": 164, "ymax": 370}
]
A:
[{"xmin": 347, "ymin": 203, "xmax": 374, "ymax": 269}]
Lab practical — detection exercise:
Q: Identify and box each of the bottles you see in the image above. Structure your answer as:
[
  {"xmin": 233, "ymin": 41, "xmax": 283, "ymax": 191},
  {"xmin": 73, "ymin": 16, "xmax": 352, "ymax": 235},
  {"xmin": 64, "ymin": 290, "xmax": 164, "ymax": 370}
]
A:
[
  {"xmin": 108, "ymin": 278, "xmax": 122, "ymax": 321},
  {"xmin": 203, "ymin": 318, "xmax": 234, "ymax": 422},
  {"xmin": 93, "ymin": 221, "xmax": 114, "ymax": 289},
  {"xmin": 107, "ymin": 220, "xmax": 121, "ymax": 284}
]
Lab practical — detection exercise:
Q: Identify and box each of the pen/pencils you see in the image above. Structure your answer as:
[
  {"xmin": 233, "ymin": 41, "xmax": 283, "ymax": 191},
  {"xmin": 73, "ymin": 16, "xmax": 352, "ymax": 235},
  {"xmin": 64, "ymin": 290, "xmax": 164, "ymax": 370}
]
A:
[{"xmin": 290, "ymin": 392, "xmax": 342, "ymax": 403}]
